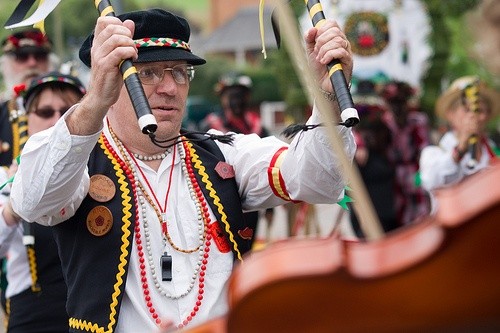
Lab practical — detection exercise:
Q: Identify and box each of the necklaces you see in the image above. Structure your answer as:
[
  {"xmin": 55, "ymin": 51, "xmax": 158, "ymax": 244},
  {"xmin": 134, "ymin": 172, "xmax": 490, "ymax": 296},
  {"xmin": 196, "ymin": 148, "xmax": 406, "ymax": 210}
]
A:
[{"xmin": 102, "ymin": 113, "xmax": 211, "ymax": 331}]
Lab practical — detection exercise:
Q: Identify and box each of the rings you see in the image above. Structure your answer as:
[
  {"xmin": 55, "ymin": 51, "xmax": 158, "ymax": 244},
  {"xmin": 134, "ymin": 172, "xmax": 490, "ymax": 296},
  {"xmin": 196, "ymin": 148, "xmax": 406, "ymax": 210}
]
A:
[{"xmin": 342, "ymin": 40, "xmax": 349, "ymax": 49}]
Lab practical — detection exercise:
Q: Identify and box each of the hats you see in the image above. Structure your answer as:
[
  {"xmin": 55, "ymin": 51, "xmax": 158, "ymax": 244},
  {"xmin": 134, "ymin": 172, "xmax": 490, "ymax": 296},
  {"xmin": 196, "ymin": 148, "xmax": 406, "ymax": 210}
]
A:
[
  {"xmin": 435, "ymin": 74, "xmax": 500, "ymax": 120},
  {"xmin": 1, "ymin": 26, "xmax": 55, "ymax": 56},
  {"xmin": 212, "ymin": 73, "xmax": 251, "ymax": 95},
  {"xmin": 78, "ymin": 9, "xmax": 207, "ymax": 69},
  {"xmin": 21, "ymin": 70, "xmax": 88, "ymax": 107}
]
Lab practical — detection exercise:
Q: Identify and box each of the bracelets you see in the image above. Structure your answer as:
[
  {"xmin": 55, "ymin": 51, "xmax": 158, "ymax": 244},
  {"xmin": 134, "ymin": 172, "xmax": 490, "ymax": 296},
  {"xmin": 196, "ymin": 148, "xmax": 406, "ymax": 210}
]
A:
[{"xmin": 314, "ymin": 76, "xmax": 353, "ymax": 104}]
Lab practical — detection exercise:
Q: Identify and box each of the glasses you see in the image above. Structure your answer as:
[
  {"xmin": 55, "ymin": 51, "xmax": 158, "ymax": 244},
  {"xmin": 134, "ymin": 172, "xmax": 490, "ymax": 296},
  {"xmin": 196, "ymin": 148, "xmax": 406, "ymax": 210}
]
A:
[
  {"xmin": 9, "ymin": 49, "xmax": 50, "ymax": 64},
  {"xmin": 135, "ymin": 64, "xmax": 199, "ymax": 86},
  {"xmin": 30, "ymin": 105, "xmax": 69, "ymax": 118}
]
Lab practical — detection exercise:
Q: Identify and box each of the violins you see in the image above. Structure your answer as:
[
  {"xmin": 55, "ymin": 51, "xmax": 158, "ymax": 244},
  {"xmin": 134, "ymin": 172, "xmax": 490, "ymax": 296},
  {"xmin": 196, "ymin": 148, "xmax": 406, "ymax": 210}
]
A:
[{"xmin": 182, "ymin": 161, "xmax": 500, "ymax": 333}]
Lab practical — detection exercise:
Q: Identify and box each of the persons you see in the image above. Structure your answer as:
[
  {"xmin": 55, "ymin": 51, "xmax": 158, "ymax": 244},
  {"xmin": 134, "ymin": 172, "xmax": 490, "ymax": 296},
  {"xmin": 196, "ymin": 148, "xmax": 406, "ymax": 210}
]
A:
[
  {"xmin": 0, "ymin": 71, "xmax": 86, "ymax": 333},
  {"xmin": 10, "ymin": 8, "xmax": 357, "ymax": 333},
  {"xmin": 181, "ymin": 75, "xmax": 500, "ymax": 257},
  {"xmin": 0, "ymin": 27, "xmax": 55, "ymax": 167}
]
